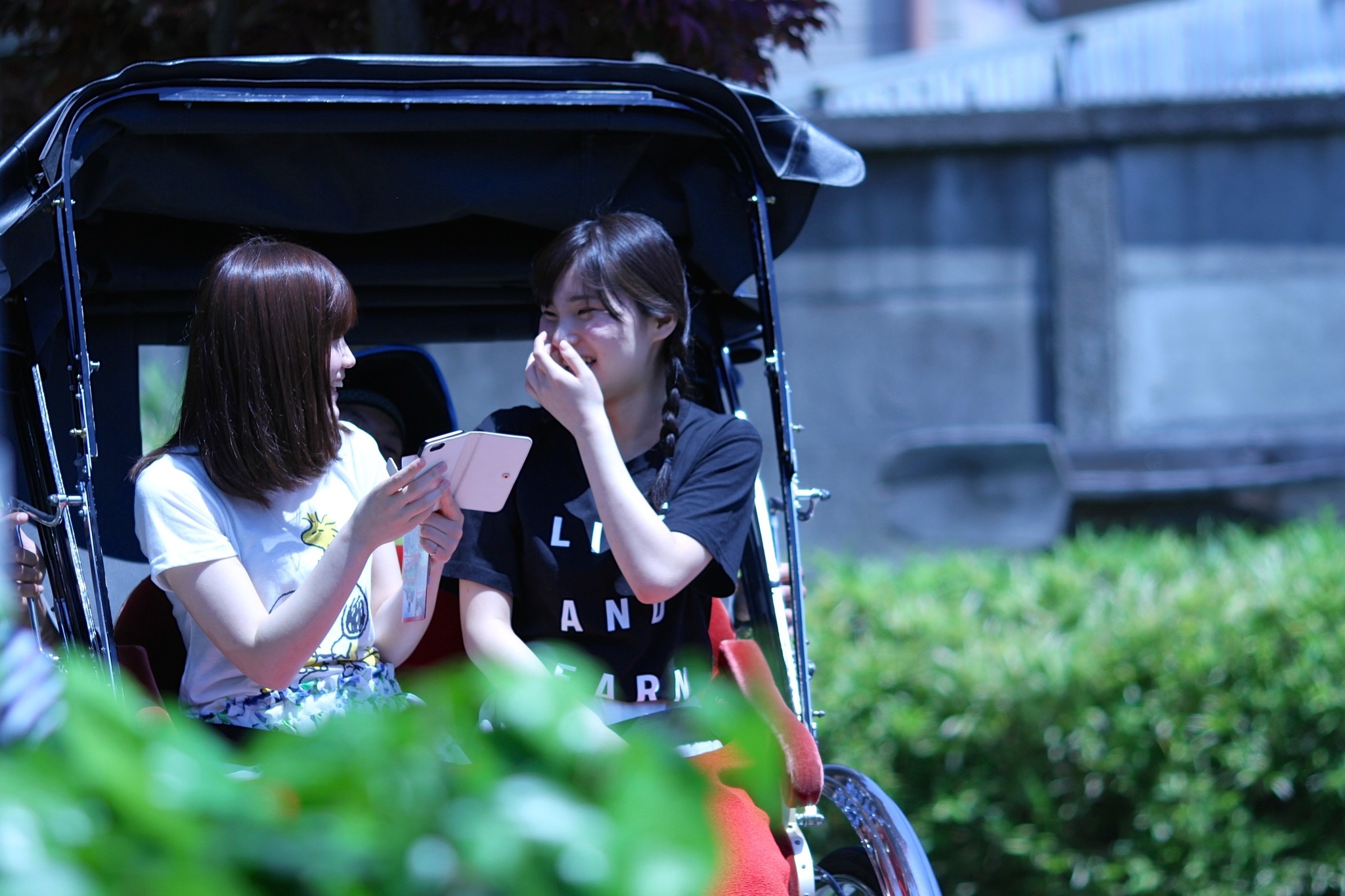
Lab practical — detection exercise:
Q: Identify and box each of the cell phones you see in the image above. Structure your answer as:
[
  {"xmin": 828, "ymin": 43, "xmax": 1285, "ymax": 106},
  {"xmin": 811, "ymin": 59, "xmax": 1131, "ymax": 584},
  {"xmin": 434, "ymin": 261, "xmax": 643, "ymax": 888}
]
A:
[{"xmin": 408, "ymin": 429, "xmax": 464, "ymax": 513}]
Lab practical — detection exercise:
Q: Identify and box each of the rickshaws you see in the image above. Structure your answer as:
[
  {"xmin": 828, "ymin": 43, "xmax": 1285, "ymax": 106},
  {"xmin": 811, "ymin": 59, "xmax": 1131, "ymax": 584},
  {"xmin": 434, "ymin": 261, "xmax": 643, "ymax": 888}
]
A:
[{"xmin": 0, "ymin": 49, "xmax": 942, "ymax": 896}]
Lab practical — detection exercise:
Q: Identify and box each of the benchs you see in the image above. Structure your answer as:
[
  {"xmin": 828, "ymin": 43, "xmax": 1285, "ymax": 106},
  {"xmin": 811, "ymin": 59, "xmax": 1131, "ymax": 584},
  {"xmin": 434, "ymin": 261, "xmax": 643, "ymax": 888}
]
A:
[{"xmin": 115, "ymin": 572, "xmax": 470, "ymax": 763}]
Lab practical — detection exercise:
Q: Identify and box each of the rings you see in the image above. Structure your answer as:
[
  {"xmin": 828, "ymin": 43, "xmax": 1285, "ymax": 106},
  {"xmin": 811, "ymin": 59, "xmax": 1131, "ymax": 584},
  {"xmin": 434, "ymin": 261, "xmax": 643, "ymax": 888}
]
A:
[{"xmin": 429, "ymin": 544, "xmax": 440, "ymax": 556}]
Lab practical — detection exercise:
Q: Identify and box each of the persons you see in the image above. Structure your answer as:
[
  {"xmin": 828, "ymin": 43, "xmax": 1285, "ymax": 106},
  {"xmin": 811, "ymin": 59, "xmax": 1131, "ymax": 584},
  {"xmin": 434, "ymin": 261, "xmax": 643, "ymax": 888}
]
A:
[
  {"xmin": 434, "ymin": 212, "xmax": 803, "ymax": 896},
  {"xmin": 0, "ymin": 237, "xmax": 463, "ymax": 778}
]
